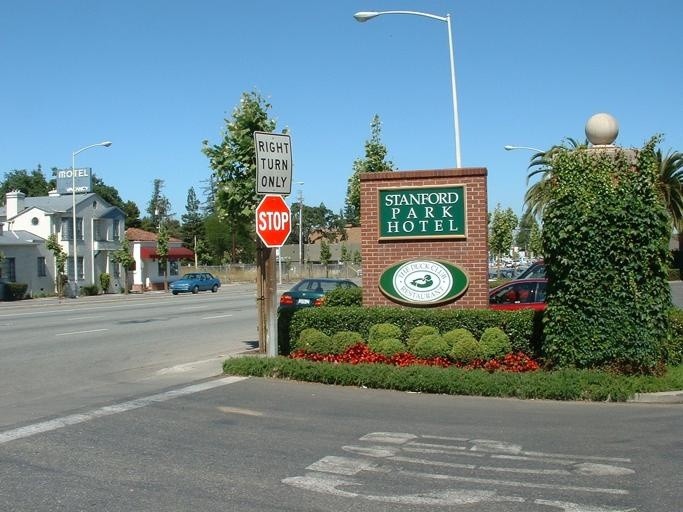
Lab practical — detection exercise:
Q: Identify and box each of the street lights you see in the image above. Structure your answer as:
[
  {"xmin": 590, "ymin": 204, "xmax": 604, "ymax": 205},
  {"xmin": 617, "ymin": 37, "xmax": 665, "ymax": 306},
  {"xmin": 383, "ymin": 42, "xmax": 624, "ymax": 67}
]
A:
[
  {"xmin": 353, "ymin": 10, "xmax": 462, "ymax": 168},
  {"xmin": 71, "ymin": 141, "xmax": 112, "ymax": 298}
]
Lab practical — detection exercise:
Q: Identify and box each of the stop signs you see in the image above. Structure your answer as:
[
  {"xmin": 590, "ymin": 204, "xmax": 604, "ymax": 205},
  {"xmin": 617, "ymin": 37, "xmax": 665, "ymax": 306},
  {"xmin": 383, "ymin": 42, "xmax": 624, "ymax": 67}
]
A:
[{"xmin": 255, "ymin": 194, "xmax": 292, "ymax": 249}]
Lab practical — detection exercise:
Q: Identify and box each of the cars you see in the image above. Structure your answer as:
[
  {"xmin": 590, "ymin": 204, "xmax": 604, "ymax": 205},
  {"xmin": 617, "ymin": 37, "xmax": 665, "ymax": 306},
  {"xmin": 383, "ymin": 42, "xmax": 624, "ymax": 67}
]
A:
[
  {"xmin": 280, "ymin": 277, "xmax": 358, "ymax": 305},
  {"xmin": 169, "ymin": 272, "xmax": 220, "ymax": 295},
  {"xmin": 490, "ymin": 261, "xmax": 548, "ymax": 310}
]
